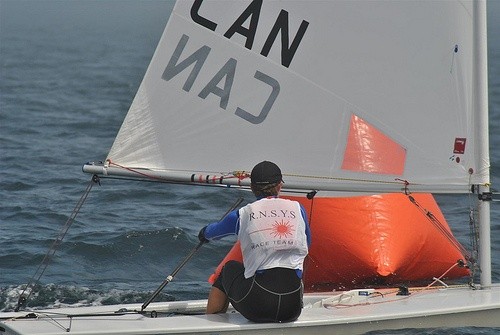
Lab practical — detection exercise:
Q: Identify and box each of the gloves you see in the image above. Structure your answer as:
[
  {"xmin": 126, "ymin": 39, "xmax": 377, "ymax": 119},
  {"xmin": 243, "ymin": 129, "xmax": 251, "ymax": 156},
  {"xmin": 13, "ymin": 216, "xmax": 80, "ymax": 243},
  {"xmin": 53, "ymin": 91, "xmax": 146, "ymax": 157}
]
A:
[{"xmin": 198, "ymin": 226, "xmax": 210, "ymax": 243}]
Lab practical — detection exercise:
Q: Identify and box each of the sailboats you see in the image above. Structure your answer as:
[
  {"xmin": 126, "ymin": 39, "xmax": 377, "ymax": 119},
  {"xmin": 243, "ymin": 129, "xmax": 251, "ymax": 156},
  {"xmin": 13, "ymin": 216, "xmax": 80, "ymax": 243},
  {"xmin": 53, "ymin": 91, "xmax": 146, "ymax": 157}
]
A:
[{"xmin": 0, "ymin": 0, "xmax": 499, "ymax": 335}]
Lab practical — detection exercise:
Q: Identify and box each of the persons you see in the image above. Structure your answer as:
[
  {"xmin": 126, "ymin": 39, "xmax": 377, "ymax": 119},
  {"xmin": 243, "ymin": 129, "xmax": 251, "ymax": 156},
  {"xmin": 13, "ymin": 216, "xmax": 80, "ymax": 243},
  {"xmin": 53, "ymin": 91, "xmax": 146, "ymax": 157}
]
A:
[{"xmin": 199, "ymin": 160, "xmax": 313, "ymax": 324}]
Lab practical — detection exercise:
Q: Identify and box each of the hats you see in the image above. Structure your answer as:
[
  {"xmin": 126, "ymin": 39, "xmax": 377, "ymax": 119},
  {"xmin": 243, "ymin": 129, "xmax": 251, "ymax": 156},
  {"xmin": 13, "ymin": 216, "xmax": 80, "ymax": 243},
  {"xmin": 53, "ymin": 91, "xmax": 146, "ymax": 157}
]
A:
[{"xmin": 251, "ymin": 161, "xmax": 285, "ymax": 185}]
